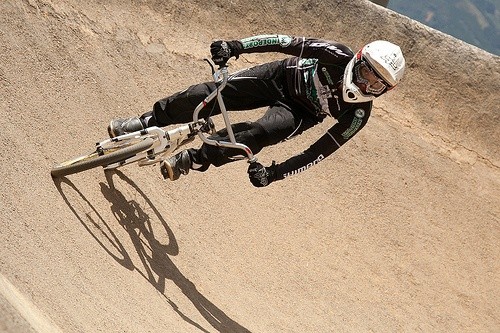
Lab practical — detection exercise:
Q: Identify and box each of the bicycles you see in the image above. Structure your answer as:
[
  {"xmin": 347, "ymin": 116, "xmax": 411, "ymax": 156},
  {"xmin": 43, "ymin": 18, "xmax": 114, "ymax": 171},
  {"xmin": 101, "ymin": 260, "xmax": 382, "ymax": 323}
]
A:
[{"xmin": 50, "ymin": 39, "xmax": 267, "ymax": 188}]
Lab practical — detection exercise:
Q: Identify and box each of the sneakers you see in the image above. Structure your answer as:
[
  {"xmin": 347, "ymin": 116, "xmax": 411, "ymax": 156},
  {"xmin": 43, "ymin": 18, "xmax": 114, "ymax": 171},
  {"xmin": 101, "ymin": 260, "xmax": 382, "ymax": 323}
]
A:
[
  {"xmin": 107, "ymin": 116, "xmax": 147, "ymax": 141},
  {"xmin": 160, "ymin": 148, "xmax": 193, "ymax": 181}
]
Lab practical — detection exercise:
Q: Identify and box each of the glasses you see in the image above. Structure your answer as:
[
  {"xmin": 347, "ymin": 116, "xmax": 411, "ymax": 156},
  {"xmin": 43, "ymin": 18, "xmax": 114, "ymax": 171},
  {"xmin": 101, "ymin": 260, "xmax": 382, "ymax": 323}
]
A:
[{"xmin": 354, "ymin": 61, "xmax": 387, "ymax": 96}]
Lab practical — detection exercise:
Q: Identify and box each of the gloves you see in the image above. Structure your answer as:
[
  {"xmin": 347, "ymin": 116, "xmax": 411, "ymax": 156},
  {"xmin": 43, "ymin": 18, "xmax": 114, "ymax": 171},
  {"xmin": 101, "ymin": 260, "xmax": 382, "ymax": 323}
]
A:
[
  {"xmin": 246, "ymin": 161, "xmax": 277, "ymax": 188},
  {"xmin": 210, "ymin": 39, "xmax": 243, "ymax": 67}
]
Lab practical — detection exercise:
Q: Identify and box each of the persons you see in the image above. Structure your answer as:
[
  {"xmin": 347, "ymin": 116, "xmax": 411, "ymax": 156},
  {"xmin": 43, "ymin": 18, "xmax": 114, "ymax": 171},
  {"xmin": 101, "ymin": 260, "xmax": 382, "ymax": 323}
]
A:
[{"xmin": 107, "ymin": 34, "xmax": 406, "ymax": 191}]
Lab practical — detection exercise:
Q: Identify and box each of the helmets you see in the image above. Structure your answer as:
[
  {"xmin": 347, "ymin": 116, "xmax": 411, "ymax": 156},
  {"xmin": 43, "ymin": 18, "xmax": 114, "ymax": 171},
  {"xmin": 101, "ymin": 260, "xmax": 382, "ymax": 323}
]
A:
[{"xmin": 342, "ymin": 40, "xmax": 406, "ymax": 104}]
